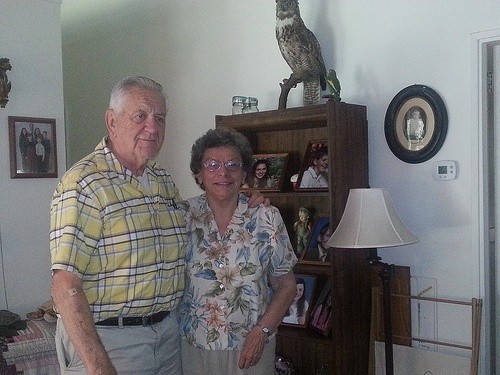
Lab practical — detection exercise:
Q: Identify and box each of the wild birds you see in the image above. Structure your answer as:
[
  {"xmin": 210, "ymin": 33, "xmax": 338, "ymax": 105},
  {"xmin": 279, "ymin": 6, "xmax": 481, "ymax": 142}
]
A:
[{"xmin": 326, "ymin": 69, "xmax": 342, "ymax": 102}]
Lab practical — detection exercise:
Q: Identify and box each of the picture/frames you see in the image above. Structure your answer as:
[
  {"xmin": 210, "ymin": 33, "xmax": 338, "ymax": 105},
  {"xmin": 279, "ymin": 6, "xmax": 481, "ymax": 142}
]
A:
[
  {"xmin": 295, "ymin": 140, "xmax": 328, "ymax": 193},
  {"xmin": 299, "ymin": 215, "xmax": 331, "ymax": 265},
  {"xmin": 240, "ymin": 153, "xmax": 289, "ymax": 193},
  {"xmin": 307, "ymin": 280, "xmax": 333, "ymax": 337},
  {"xmin": 280, "ymin": 271, "xmax": 320, "ymax": 329},
  {"xmin": 7, "ymin": 116, "xmax": 58, "ymax": 179},
  {"xmin": 384, "ymin": 84, "xmax": 448, "ymax": 163}
]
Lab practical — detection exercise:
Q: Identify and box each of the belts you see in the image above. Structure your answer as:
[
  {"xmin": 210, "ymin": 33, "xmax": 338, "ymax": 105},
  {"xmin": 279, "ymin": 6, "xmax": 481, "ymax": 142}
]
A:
[{"xmin": 95, "ymin": 310, "xmax": 171, "ymax": 327}]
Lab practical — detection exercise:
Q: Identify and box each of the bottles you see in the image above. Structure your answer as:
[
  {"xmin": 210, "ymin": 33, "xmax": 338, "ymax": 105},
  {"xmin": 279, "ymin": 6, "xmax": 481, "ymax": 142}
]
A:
[{"xmin": 231, "ymin": 96, "xmax": 259, "ymax": 115}]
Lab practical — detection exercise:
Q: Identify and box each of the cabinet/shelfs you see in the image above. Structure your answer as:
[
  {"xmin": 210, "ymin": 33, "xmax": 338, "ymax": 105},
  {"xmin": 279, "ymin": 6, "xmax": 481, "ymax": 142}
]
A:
[{"xmin": 215, "ymin": 102, "xmax": 372, "ymax": 375}]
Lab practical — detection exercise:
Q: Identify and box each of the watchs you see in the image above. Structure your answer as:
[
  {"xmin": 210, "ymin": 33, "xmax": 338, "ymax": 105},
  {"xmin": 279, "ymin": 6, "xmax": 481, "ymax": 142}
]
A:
[{"xmin": 257, "ymin": 322, "xmax": 272, "ymax": 337}]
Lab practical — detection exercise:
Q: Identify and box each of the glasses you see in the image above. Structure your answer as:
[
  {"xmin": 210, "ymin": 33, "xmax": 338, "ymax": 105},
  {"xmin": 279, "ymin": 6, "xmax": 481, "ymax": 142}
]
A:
[{"xmin": 200, "ymin": 160, "xmax": 247, "ymax": 171}]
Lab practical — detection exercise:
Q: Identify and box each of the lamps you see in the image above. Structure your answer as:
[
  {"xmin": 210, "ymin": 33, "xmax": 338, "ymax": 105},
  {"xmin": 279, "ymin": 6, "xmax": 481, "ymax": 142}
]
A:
[{"xmin": 326, "ymin": 187, "xmax": 420, "ymax": 375}]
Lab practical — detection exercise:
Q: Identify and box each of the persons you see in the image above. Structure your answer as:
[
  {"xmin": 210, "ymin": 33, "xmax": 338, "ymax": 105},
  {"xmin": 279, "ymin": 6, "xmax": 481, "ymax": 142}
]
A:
[
  {"xmin": 281, "ymin": 277, "xmax": 309, "ymax": 326},
  {"xmin": 179, "ymin": 125, "xmax": 299, "ymax": 375},
  {"xmin": 241, "ymin": 159, "xmax": 275, "ymax": 190},
  {"xmin": 309, "ymin": 222, "xmax": 331, "ymax": 262},
  {"xmin": 407, "ymin": 110, "xmax": 425, "ymax": 142},
  {"xmin": 299, "ymin": 146, "xmax": 329, "ymax": 188},
  {"xmin": 49, "ymin": 74, "xmax": 271, "ymax": 375},
  {"xmin": 293, "ymin": 206, "xmax": 312, "ymax": 257},
  {"xmin": 18, "ymin": 123, "xmax": 52, "ymax": 173},
  {"xmin": 311, "ymin": 289, "xmax": 333, "ymax": 331}
]
